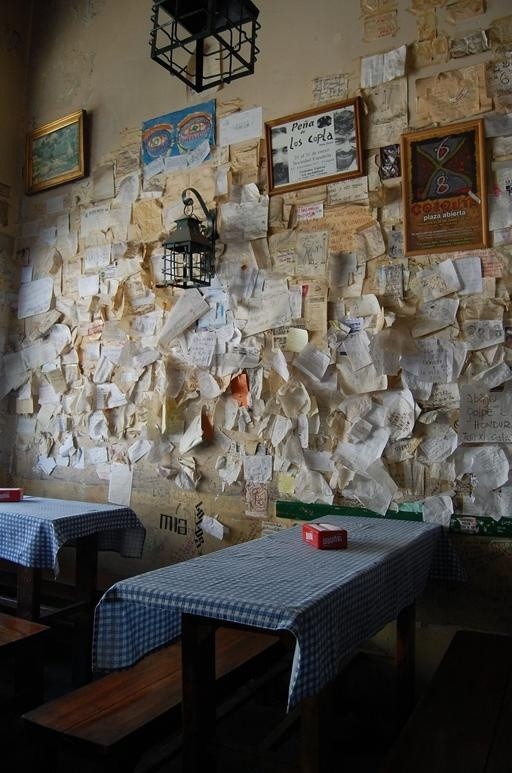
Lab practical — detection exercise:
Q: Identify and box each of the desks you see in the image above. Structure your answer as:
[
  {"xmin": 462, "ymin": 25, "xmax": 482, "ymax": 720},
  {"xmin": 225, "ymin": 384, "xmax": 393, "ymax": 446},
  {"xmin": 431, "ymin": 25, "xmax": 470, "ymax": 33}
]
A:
[
  {"xmin": 92, "ymin": 514, "xmax": 473, "ymax": 772},
  {"xmin": 0, "ymin": 494, "xmax": 474, "ymax": 773},
  {"xmin": 1, "ymin": 495, "xmax": 146, "ymax": 623}
]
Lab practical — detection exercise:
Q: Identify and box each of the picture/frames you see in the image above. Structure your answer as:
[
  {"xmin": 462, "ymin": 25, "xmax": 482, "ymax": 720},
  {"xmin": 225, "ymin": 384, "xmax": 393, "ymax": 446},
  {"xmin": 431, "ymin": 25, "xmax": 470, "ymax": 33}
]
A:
[
  {"xmin": 24, "ymin": 109, "xmax": 88, "ymax": 195},
  {"xmin": 400, "ymin": 118, "xmax": 490, "ymax": 257},
  {"xmin": 264, "ymin": 96, "xmax": 366, "ymax": 196}
]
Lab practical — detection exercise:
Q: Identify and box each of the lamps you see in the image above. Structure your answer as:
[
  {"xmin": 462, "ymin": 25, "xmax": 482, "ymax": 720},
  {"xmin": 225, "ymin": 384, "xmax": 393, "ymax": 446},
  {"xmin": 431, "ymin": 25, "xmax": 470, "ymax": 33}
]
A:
[{"xmin": 161, "ymin": 188, "xmax": 217, "ymax": 289}]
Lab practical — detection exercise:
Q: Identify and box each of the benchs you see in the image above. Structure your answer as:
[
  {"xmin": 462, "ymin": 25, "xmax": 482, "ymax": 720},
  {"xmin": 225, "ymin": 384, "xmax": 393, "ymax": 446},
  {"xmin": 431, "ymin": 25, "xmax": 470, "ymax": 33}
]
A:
[
  {"xmin": 1, "ymin": 613, "xmax": 52, "ymax": 663},
  {"xmin": 379, "ymin": 629, "xmax": 512, "ymax": 773},
  {"xmin": 21, "ymin": 628, "xmax": 288, "ymax": 772}
]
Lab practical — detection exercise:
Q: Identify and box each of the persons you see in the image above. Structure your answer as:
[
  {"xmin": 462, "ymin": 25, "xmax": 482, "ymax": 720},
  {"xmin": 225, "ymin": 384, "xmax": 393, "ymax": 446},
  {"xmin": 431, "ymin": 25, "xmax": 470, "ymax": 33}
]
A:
[
  {"xmin": 271, "ymin": 126, "xmax": 289, "ymax": 186},
  {"xmin": 333, "ymin": 109, "xmax": 357, "ymax": 172}
]
